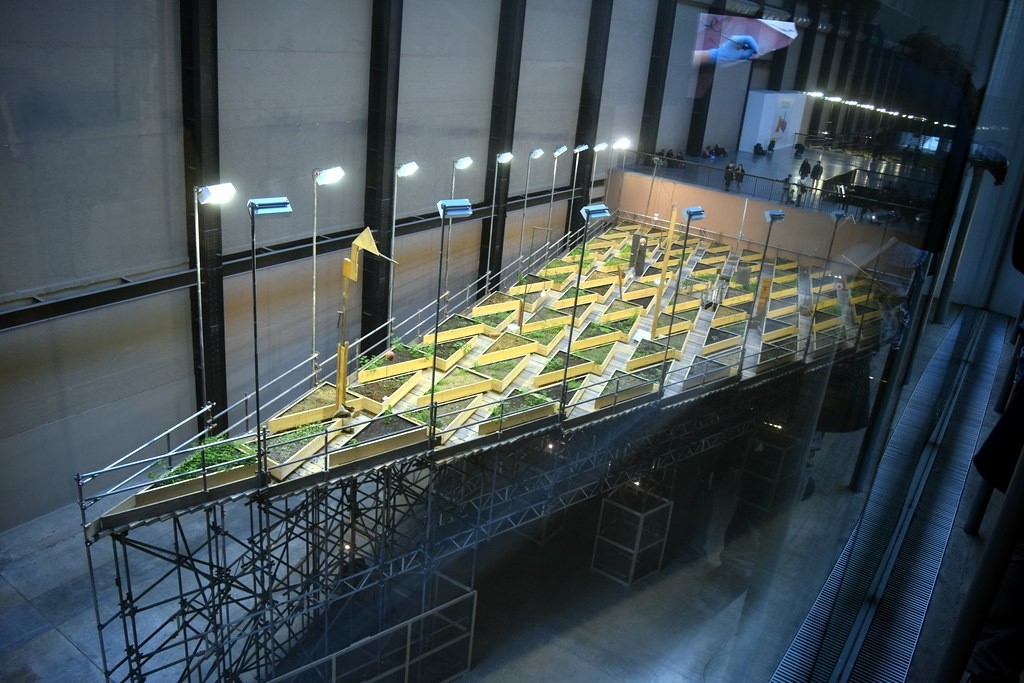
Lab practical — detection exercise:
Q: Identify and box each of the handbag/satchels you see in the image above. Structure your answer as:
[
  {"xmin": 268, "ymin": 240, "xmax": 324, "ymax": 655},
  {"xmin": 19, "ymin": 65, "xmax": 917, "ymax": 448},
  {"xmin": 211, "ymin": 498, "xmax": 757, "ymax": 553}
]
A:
[{"xmin": 801, "ymin": 185, "xmax": 808, "ymax": 193}]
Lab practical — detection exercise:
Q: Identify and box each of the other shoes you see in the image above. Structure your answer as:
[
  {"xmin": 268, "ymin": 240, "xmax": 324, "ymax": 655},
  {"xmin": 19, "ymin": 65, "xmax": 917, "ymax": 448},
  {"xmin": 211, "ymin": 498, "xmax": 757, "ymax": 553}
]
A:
[
  {"xmin": 795, "ymin": 204, "xmax": 800, "ymax": 207},
  {"xmin": 784, "ymin": 183, "xmax": 790, "ymax": 189}
]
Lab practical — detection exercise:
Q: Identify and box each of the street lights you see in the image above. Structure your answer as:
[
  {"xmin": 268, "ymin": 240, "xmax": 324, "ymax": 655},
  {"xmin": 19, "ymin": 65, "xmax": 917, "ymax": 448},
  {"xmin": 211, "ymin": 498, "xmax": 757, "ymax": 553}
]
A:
[
  {"xmin": 443, "ymin": 156, "xmax": 474, "ymax": 318},
  {"xmin": 590, "ymin": 142, "xmax": 610, "ymax": 205},
  {"xmin": 246, "ymin": 195, "xmax": 292, "ymax": 474},
  {"xmin": 312, "ymin": 165, "xmax": 345, "ymax": 390},
  {"xmin": 567, "ymin": 145, "xmax": 590, "ymax": 251},
  {"xmin": 657, "ymin": 204, "xmax": 708, "ymax": 401},
  {"xmin": 853, "ymin": 210, "xmax": 898, "ymax": 347},
  {"xmin": 545, "ymin": 145, "xmax": 569, "ymax": 266},
  {"xmin": 804, "ymin": 209, "xmax": 847, "ymax": 363},
  {"xmin": 427, "ymin": 196, "xmax": 474, "ymax": 452},
  {"xmin": 556, "ymin": 201, "xmax": 611, "ymax": 425},
  {"xmin": 518, "ymin": 148, "xmax": 544, "ymax": 277},
  {"xmin": 737, "ymin": 208, "xmax": 786, "ymax": 377},
  {"xmin": 387, "ymin": 161, "xmax": 420, "ymax": 352},
  {"xmin": 485, "ymin": 151, "xmax": 514, "ymax": 296},
  {"xmin": 193, "ymin": 181, "xmax": 239, "ymax": 443},
  {"xmin": 605, "ymin": 138, "xmax": 631, "ymax": 207}
]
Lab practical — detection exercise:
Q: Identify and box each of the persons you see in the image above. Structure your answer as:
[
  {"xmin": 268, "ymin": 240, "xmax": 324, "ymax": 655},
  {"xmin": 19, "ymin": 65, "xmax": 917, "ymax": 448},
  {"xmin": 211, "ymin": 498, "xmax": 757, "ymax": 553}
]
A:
[
  {"xmin": 693, "ymin": 35, "xmax": 758, "ymax": 67},
  {"xmin": 653, "ymin": 140, "xmax": 922, "ymax": 207}
]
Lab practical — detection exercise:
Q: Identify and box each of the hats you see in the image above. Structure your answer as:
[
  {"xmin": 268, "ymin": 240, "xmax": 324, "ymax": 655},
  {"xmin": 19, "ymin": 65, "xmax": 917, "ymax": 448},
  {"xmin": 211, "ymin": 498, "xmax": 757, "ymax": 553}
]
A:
[{"xmin": 817, "ymin": 159, "xmax": 822, "ymax": 164}]
